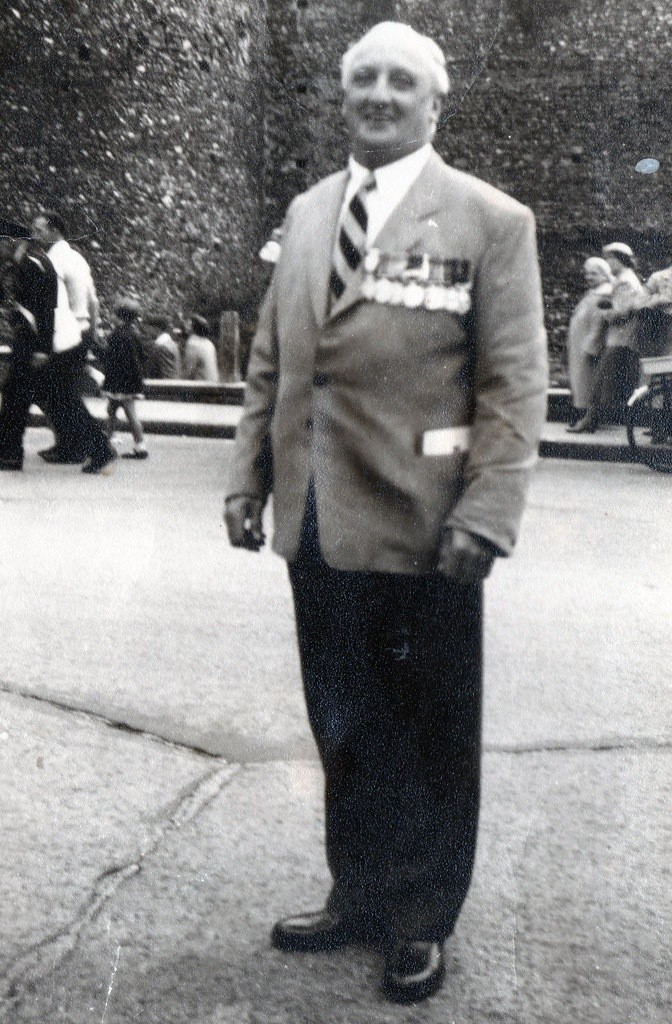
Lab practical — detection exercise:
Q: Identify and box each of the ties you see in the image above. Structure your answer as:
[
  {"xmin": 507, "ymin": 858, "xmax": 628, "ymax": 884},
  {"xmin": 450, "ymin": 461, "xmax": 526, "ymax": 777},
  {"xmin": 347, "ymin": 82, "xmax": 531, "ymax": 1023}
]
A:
[{"xmin": 327, "ymin": 175, "xmax": 375, "ymax": 309}]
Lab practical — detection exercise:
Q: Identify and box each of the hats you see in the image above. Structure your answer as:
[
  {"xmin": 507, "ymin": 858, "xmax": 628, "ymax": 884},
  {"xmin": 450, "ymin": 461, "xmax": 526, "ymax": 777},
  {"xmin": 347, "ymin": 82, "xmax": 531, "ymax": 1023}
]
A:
[
  {"xmin": 0, "ymin": 216, "xmax": 32, "ymax": 241},
  {"xmin": 603, "ymin": 242, "xmax": 634, "ymax": 258}
]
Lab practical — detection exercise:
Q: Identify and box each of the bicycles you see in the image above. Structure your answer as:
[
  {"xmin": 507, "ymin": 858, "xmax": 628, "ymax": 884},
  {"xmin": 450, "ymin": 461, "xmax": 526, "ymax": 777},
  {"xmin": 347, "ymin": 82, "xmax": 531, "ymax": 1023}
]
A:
[{"xmin": 625, "ymin": 369, "xmax": 672, "ymax": 473}]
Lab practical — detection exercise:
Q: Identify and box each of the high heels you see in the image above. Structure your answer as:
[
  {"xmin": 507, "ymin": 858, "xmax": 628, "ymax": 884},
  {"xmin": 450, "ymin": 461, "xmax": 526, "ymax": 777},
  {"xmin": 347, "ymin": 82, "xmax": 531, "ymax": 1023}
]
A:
[{"xmin": 566, "ymin": 422, "xmax": 595, "ymax": 434}]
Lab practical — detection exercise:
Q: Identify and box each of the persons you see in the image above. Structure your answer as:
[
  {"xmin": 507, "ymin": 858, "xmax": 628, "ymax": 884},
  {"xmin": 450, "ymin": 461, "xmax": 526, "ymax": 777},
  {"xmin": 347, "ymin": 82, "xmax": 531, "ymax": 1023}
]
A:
[
  {"xmin": 222, "ymin": 21, "xmax": 548, "ymax": 1005},
  {"xmin": 0, "ymin": 211, "xmax": 218, "ymax": 474},
  {"xmin": 567, "ymin": 241, "xmax": 643, "ymax": 434}
]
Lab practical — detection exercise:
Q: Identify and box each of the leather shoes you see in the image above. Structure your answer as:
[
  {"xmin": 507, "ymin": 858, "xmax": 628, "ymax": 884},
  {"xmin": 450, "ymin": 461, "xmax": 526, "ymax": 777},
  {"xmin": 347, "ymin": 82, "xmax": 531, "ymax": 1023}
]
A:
[
  {"xmin": 382, "ymin": 933, "xmax": 446, "ymax": 1002},
  {"xmin": 271, "ymin": 906, "xmax": 375, "ymax": 950}
]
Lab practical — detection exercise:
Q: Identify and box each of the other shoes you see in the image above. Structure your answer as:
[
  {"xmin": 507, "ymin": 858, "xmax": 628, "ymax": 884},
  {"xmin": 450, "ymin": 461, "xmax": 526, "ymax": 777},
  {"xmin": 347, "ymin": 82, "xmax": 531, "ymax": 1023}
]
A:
[
  {"xmin": 122, "ymin": 447, "xmax": 148, "ymax": 458},
  {"xmin": 39, "ymin": 448, "xmax": 83, "ymax": 465},
  {"xmin": 85, "ymin": 448, "xmax": 115, "ymax": 471},
  {"xmin": 1, "ymin": 456, "xmax": 23, "ymax": 471}
]
